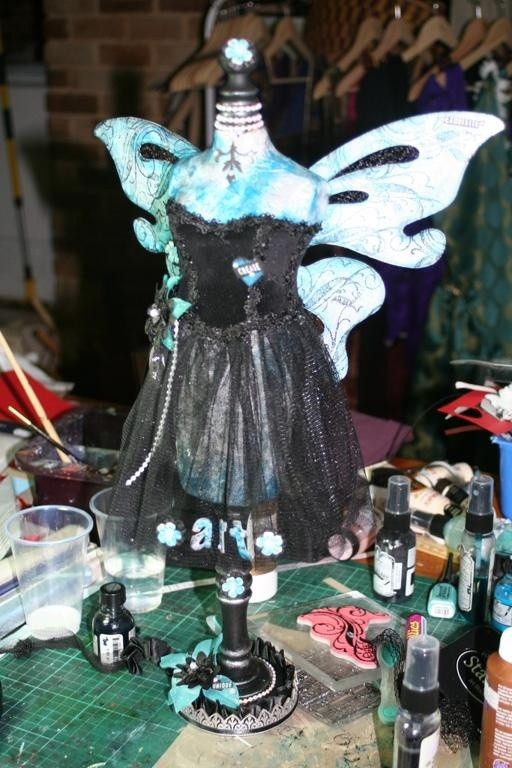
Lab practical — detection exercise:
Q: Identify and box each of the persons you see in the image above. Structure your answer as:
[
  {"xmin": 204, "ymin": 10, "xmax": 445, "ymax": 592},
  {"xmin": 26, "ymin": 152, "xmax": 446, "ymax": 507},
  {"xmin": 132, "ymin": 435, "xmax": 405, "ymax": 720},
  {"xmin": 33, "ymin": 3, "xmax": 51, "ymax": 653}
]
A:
[{"xmin": 91, "ymin": 37, "xmax": 376, "ymax": 582}]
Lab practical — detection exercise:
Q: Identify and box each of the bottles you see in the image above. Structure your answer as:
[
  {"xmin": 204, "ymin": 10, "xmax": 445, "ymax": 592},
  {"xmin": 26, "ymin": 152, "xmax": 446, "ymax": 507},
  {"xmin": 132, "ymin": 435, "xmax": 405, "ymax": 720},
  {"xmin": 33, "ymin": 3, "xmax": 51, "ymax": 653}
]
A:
[
  {"xmin": 370, "ymin": 469, "xmax": 420, "ymax": 601},
  {"xmin": 91, "ymin": 579, "xmax": 140, "ymax": 673},
  {"xmin": 456, "ymin": 474, "xmax": 498, "ymax": 623},
  {"xmin": 393, "ymin": 633, "xmax": 441, "ymax": 767}
]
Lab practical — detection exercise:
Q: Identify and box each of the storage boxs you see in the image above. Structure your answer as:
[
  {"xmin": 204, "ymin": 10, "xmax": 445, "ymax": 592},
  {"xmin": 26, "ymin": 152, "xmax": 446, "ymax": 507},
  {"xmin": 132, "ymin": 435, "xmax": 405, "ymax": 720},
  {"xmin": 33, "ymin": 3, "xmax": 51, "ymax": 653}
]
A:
[{"xmin": 14, "ymin": 409, "xmax": 219, "ymax": 569}]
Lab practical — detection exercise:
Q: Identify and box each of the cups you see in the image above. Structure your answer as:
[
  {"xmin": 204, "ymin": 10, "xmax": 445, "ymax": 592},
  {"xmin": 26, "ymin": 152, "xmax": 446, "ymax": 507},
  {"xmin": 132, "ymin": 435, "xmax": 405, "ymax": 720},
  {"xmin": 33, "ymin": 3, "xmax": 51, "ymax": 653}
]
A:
[
  {"xmin": 5, "ymin": 503, "xmax": 95, "ymax": 644},
  {"xmin": 90, "ymin": 485, "xmax": 177, "ymax": 616}
]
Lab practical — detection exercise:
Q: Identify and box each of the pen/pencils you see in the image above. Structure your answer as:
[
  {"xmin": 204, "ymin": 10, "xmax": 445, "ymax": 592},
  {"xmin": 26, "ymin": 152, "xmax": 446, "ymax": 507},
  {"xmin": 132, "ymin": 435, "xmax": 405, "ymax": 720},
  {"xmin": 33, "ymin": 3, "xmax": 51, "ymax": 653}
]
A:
[{"xmin": 1, "ymin": 331, "xmax": 86, "ymax": 463}]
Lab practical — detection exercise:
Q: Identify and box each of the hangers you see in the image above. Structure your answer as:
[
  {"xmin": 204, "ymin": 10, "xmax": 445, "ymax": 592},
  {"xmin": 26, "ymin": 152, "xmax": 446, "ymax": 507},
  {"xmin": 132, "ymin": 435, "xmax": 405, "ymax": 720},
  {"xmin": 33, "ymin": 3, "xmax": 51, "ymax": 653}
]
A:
[{"xmin": 166, "ymin": 2, "xmax": 510, "ymax": 153}]
